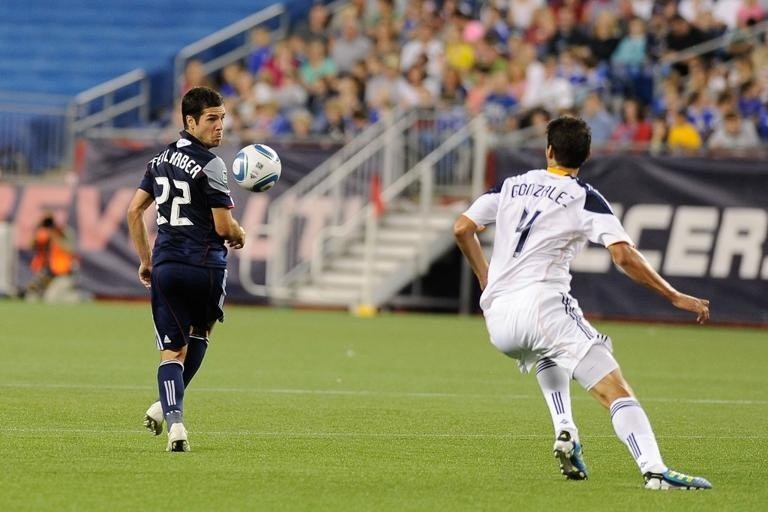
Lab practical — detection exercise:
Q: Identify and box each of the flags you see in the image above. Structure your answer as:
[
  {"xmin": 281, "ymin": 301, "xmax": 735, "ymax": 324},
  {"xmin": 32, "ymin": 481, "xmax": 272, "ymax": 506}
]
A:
[{"xmin": 370, "ymin": 176, "xmax": 386, "ymax": 214}]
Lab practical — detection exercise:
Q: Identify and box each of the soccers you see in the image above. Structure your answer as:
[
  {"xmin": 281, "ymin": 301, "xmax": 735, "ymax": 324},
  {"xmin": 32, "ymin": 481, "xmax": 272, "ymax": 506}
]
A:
[{"xmin": 232, "ymin": 144, "xmax": 281, "ymax": 192}]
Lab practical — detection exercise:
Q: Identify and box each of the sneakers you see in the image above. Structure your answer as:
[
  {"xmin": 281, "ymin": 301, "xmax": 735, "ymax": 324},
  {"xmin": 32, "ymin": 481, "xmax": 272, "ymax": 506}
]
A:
[
  {"xmin": 144, "ymin": 401, "xmax": 165, "ymax": 435},
  {"xmin": 553, "ymin": 429, "xmax": 587, "ymax": 480},
  {"xmin": 167, "ymin": 422, "xmax": 190, "ymax": 452},
  {"xmin": 642, "ymin": 470, "xmax": 712, "ymax": 489}
]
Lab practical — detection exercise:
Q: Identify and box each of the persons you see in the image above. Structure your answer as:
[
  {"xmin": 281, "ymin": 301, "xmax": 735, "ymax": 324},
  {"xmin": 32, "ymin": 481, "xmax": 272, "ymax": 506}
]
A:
[
  {"xmin": 126, "ymin": 85, "xmax": 244, "ymax": 451},
  {"xmin": 452, "ymin": 115, "xmax": 712, "ymax": 491},
  {"xmin": 167, "ymin": 0, "xmax": 767, "ymax": 155},
  {"xmin": 22, "ymin": 211, "xmax": 78, "ymax": 299}
]
